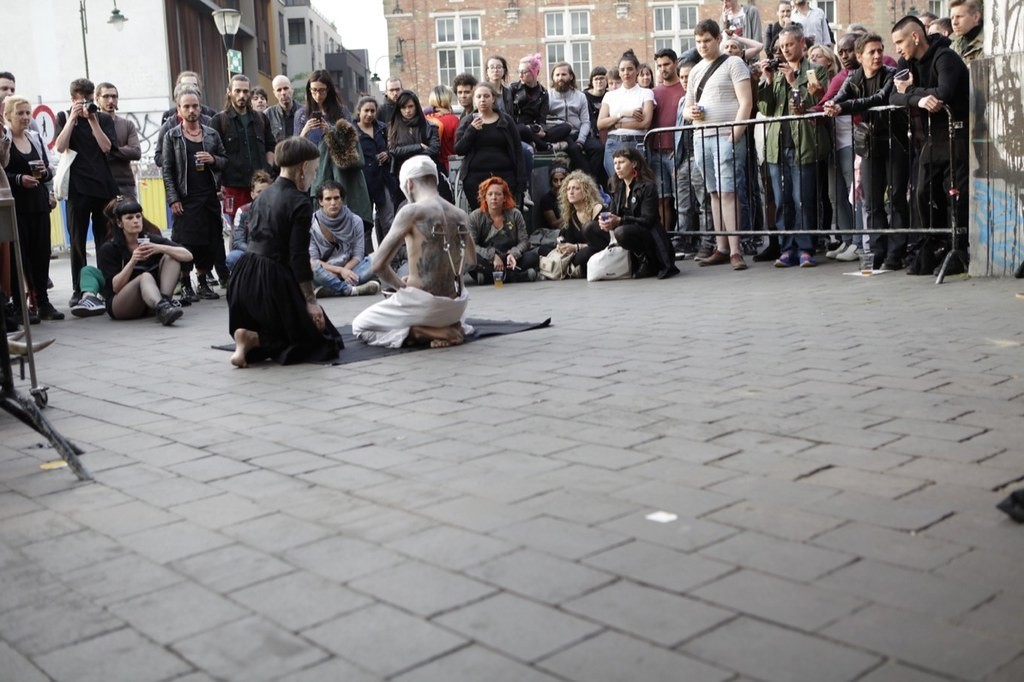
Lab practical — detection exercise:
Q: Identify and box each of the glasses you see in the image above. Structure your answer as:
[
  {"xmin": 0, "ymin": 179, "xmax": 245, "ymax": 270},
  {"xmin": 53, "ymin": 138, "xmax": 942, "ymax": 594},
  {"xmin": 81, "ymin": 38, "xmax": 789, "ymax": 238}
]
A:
[
  {"xmin": 771, "ymin": 47, "xmax": 782, "ymax": 54},
  {"xmin": 517, "ymin": 70, "xmax": 531, "ymax": 75},
  {"xmin": 310, "ymin": 88, "xmax": 328, "ymax": 94},
  {"xmin": 486, "ymin": 65, "xmax": 502, "ymax": 71}
]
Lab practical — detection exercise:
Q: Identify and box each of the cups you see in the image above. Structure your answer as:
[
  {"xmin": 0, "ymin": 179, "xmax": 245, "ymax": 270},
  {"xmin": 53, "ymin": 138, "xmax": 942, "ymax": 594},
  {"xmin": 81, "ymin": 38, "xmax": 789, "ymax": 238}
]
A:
[
  {"xmin": 224, "ymin": 195, "xmax": 234, "ymax": 213},
  {"xmin": 600, "ymin": 212, "xmax": 611, "ymax": 222},
  {"xmin": 473, "ymin": 113, "xmax": 483, "ymax": 128},
  {"xmin": 137, "ymin": 237, "xmax": 150, "ymax": 259},
  {"xmin": 694, "ymin": 103, "xmax": 704, "ymax": 119},
  {"xmin": 194, "ymin": 155, "xmax": 204, "ymax": 171},
  {"xmin": 493, "ymin": 272, "xmax": 503, "ymax": 287},
  {"xmin": 28, "ymin": 160, "xmax": 42, "ymax": 179},
  {"xmin": 893, "ymin": 69, "xmax": 910, "ymax": 81}
]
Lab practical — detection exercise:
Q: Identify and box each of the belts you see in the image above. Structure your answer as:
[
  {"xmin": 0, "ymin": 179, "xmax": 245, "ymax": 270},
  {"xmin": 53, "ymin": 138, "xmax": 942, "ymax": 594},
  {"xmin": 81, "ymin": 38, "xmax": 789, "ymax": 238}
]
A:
[
  {"xmin": 653, "ymin": 148, "xmax": 672, "ymax": 155},
  {"xmin": 607, "ymin": 134, "xmax": 645, "ymax": 143}
]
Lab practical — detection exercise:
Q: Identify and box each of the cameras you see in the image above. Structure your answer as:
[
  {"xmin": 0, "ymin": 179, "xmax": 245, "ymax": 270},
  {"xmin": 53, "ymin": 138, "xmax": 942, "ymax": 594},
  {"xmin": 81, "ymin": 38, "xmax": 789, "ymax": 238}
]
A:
[
  {"xmin": 80, "ymin": 98, "xmax": 97, "ymax": 113},
  {"xmin": 764, "ymin": 55, "xmax": 784, "ymax": 73},
  {"xmin": 530, "ymin": 125, "xmax": 540, "ymax": 133}
]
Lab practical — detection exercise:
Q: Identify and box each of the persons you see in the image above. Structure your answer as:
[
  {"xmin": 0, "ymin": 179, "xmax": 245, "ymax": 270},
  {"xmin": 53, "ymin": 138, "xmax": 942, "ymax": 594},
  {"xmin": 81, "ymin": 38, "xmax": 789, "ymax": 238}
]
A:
[{"xmin": 0, "ymin": 0, "xmax": 984, "ymax": 367}]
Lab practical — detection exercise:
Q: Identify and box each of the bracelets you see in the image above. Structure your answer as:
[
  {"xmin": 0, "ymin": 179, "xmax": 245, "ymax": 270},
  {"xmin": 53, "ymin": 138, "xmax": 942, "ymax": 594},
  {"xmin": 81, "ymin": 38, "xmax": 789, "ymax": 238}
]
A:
[
  {"xmin": 576, "ymin": 243, "xmax": 581, "ymax": 252},
  {"xmin": 620, "ymin": 123, "xmax": 622, "ymax": 128},
  {"xmin": 618, "ymin": 113, "xmax": 622, "ymax": 119}
]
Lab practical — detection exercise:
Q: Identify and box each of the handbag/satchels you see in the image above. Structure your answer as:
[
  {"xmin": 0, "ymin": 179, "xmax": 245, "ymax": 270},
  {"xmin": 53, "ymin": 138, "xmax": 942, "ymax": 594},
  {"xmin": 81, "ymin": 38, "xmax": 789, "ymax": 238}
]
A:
[
  {"xmin": 53, "ymin": 148, "xmax": 78, "ymax": 201},
  {"xmin": 676, "ymin": 120, "xmax": 694, "ymax": 167},
  {"xmin": 539, "ymin": 243, "xmax": 577, "ymax": 281},
  {"xmin": 528, "ymin": 228, "xmax": 561, "ymax": 249},
  {"xmin": 854, "ymin": 121, "xmax": 877, "ymax": 159},
  {"xmin": 586, "ymin": 230, "xmax": 631, "ymax": 281}
]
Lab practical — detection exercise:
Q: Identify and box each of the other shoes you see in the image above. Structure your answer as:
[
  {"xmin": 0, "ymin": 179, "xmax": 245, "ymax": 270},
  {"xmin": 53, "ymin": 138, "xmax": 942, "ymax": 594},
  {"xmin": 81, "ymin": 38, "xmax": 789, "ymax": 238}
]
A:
[
  {"xmin": 478, "ymin": 269, "xmax": 493, "ymax": 285},
  {"xmin": 313, "ymin": 285, "xmax": 334, "ymax": 298},
  {"xmin": 669, "ymin": 235, "xmax": 969, "ymax": 275},
  {"xmin": 514, "ymin": 268, "xmax": 536, "ymax": 283},
  {"xmin": 524, "ymin": 206, "xmax": 529, "ymax": 212},
  {"xmin": 4, "ymin": 278, "xmax": 65, "ymax": 332},
  {"xmin": 157, "ymin": 270, "xmax": 229, "ymax": 325},
  {"xmin": 548, "ymin": 141, "xmax": 568, "ymax": 152},
  {"xmin": 524, "ymin": 193, "xmax": 534, "ymax": 205},
  {"xmin": 69, "ymin": 292, "xmax": 82, "ymax": 307},
  {"xmin": 570, "ymin": 264, "xmax": 581, "ymax": 277},
  {"xmin": 355, "ymin": 280, "xmax": 380, "ymax": 296},
  {"xmin": 633, "ymin": 257, "xmax": 655, "ymax": 278}
]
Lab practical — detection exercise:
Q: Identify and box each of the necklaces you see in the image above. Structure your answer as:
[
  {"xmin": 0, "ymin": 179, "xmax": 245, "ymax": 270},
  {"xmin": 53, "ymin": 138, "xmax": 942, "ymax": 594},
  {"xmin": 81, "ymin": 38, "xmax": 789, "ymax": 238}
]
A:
[{"xmin": 182, "ymin": 124, "xmax": 203, "ymax": 137}]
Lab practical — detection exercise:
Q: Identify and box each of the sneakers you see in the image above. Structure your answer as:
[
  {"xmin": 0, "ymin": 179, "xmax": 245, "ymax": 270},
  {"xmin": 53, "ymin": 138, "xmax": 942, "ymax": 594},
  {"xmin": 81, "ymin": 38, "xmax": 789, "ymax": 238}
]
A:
[{"xmin": 71, "ymin": 294, "xmax": 106, "ymax": 317}]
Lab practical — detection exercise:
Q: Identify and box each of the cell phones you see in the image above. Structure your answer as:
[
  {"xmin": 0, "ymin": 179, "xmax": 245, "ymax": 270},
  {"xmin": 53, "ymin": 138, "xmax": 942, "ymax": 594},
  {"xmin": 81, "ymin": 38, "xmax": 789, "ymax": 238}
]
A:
[
  {"xmin": 473, "ymin": 113, "xmax": 482, "ymax": 129},
  {"xmin": 312, "ymin": 112, "xmax": 322, "ymax": 122},
  {"xmin": 805, "ymin": 69, "xmax": 819, "ymax": 86},
  {"xmin": 792, "ymin": 89, "xmax": 800, "ymax": 108}
]
